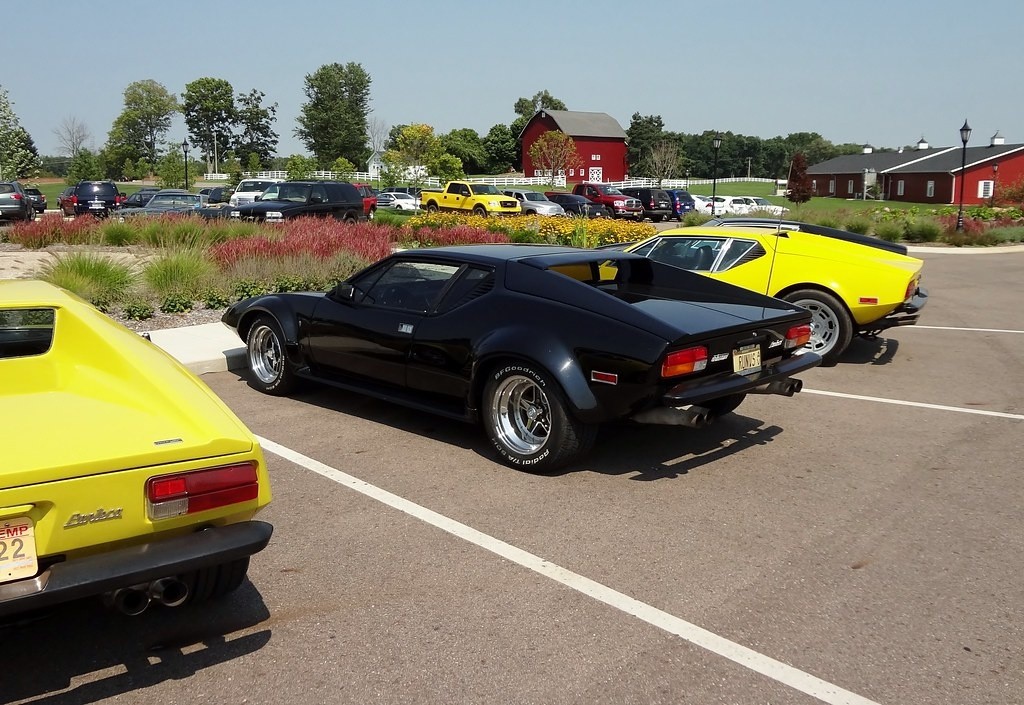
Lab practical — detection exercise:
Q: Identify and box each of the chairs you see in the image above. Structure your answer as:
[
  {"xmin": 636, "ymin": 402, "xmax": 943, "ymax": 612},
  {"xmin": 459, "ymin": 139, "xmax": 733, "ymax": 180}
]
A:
[{"xmin": 693, "ymin": 245, "xmax": 713, "ymax": 270}]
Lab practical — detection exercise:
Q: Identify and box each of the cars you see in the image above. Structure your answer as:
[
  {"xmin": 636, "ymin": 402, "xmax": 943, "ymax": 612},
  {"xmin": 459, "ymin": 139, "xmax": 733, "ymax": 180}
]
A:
[
  {"xmin": 546, "ymin": 194, "xmax": 609, "ymax": 219},
  {"xmin": 499, "ymin": 189, "xmax": 566, "ymax": 217},
  {"xmin": 376, "ymin": 192, "xmax": 421, "ymax": 210},
  {"xmin": 121, "ymin": 189, "xmax": 158, "ymax": 208},
  {"xmin": 56, "ymin": 186, "xmax": 75, "ymax": 218},
  {"xmin": 707, "ymin": 196, "xmax": 749, "ymax": 216},
  {"xmin": 198, "ymin": 178, "xmax": 365, "ymax": 222},
  {"xmin": 690, "ymin": 194, "xmax": 713, "ymax": 215},
  {"xmin": 663, "ymin": 189, "xmax": 695, "ymax": 222},
  {"xmin": 737, "ymin": 196, "xmax": 790, "ymax": 216},
  {"xmin": 29, "ymin": 188, "xmax": 48, "ymax": 214},
  {"xmin": 547, "ymin": 226, "xmax": 925, "ymax": 366}
]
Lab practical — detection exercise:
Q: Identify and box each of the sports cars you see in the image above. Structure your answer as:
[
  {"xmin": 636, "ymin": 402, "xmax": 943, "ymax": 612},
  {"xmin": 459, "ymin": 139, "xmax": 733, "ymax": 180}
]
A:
[
  {"xmin": 0, "ymin": 278, "xmax": 273, "ymax": 620},
  {"xmin": 220, "ymin": 243, "xmax": 823, "ymax": 471},
  {"xmin": 592, "ymin": 217, "xmax": 930, "ymax": 315}
]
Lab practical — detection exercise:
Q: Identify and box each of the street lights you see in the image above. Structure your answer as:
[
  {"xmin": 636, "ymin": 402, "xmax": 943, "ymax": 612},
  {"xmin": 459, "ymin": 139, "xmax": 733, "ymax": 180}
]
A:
[
  {"xmin": 956, "ymin": 119, "xmax": 972, "ymax": 233},
  {"xmin": 685, "ymin": 170, "xmax": 689, "ymax": 191},
  {"xmin": 990, "ymin": 159, "xmax": 999, "ymax": 208},
  {"xmin": 181, "ymin": 138, "xmax": 190, "ymax": 190},
  {"xmin": 214, "ymin": 130, "xmax": 218, "ymax": 174},
  {"xmin": 711, "ymin": 131, "xmax": 723, "ymax": 215}
]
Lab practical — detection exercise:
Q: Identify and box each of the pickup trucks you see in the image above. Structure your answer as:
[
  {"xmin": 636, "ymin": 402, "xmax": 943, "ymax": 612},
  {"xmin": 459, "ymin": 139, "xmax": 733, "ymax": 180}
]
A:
[
  {"xmin": 419, "ymin": 181, "xmax": 522, "ymax": 219},
  {"xmin": 545, "ymin": 183, "xmax": 644, "ymax": 220}
]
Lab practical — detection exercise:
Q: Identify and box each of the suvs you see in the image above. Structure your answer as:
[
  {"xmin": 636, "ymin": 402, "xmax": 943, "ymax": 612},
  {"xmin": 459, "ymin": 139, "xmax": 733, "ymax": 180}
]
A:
[
  {"xmin": 617, "ymin": 187, "xmax": 672, "ymax": 222},
  {"xmin": 111, "ymin": 192, "xmax": 208, "ymax": 219},
  {"xmin": 352, "ymin": 182, "xmax": 378, "ymax": 221},
  {"xmin": 0, "ymin": 181, "xmax": 36, "ymax": 223},
  {"xmin": 72, "ymin": 177, "xmax": 126, "ymax": 218}
]
